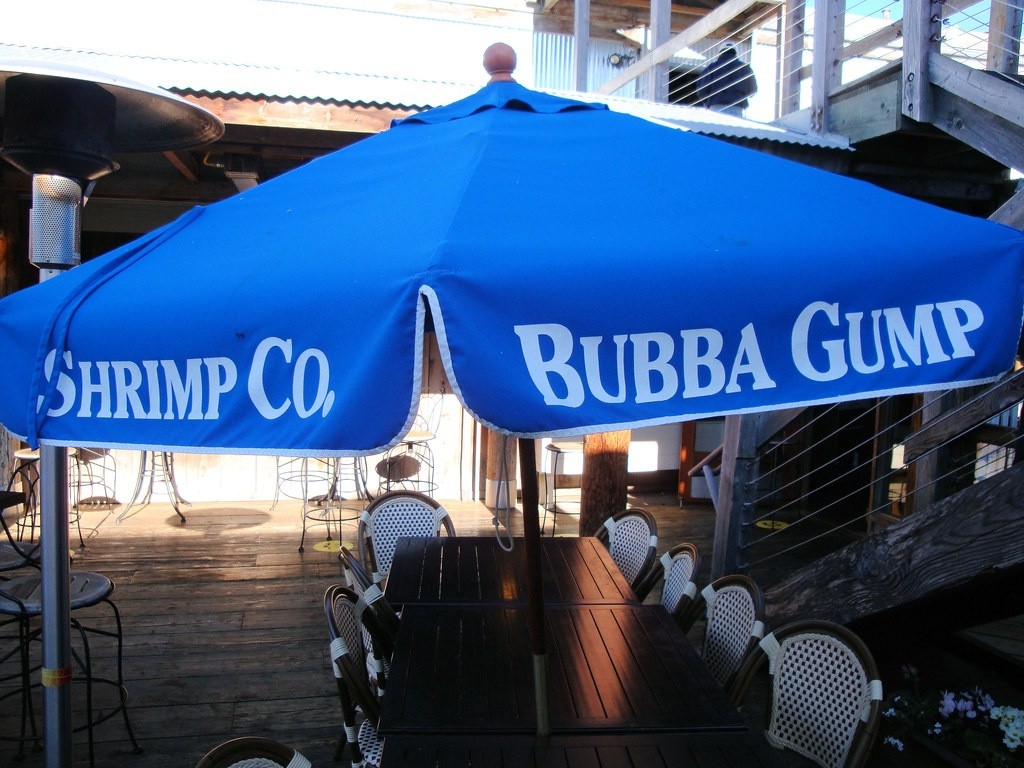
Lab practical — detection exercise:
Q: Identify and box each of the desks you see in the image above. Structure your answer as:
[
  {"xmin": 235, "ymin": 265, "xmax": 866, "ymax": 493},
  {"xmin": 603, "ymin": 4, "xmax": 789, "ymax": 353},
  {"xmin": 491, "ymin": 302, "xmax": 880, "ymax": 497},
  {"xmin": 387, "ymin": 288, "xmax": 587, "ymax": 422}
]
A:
[
  {"xmin": 382, "ymin": 536, "xmax": 640, "ymax": 607},
  {"xmin": 379, "ymin": 732, "xmax": 788, "ymax": 768},
  {"xmin": 0, "ymin": 490, "xmax": 41, "ymax": 571},
  {"xmin": 376, "ymin": 603, "xmax": 751, "ymax": 742}
]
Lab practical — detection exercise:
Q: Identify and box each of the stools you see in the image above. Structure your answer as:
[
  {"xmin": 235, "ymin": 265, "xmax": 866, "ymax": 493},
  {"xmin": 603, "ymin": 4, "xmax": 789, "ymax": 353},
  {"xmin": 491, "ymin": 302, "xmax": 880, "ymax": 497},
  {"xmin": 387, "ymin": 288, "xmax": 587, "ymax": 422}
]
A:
[
  {"xmin": 0, "ymin": 570, "xmax": 143, "ymax": 768},
  {"xmin": 12, "ymin": 386, "xmax": 444, "ymax": 554},
  {"xmin": 540, "ymin": 441, "xmax": 583, "ymax": 536}
]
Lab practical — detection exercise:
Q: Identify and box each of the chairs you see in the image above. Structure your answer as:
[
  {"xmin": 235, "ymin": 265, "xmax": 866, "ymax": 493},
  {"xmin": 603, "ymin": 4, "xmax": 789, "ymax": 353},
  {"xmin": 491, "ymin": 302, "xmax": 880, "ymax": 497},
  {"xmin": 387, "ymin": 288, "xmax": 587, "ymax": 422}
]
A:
[{"xmin": 197, "ymin": 490, "xmax": 884, "ymax": 768}]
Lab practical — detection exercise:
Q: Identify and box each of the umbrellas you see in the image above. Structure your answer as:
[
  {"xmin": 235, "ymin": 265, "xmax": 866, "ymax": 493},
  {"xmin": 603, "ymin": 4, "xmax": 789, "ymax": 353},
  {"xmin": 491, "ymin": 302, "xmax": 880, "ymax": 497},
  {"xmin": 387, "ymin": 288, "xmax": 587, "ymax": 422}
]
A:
[{"xmin": 0, "ymin": 40, "xmax": 1024, "ymax": 737}]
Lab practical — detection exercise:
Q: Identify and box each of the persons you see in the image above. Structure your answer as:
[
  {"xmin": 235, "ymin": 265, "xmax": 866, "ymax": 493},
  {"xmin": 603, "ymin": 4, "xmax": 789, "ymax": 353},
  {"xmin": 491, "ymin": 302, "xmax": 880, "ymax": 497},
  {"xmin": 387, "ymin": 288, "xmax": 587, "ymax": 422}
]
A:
[{"xmin": 697, "ymin": 42, "xmax": 757, "ymax": 117}]
